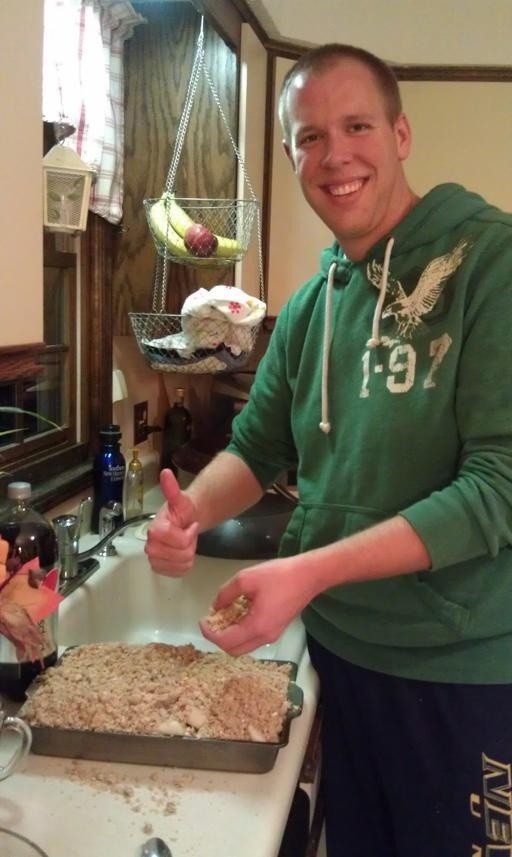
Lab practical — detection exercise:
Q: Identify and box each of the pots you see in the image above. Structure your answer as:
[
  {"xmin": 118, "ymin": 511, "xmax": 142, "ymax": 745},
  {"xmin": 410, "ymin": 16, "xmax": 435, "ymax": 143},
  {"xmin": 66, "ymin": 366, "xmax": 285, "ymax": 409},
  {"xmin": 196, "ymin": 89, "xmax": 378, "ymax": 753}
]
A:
[{"xmin": 195, "ymin": 492, "xmax": 298, "ymax": 560}]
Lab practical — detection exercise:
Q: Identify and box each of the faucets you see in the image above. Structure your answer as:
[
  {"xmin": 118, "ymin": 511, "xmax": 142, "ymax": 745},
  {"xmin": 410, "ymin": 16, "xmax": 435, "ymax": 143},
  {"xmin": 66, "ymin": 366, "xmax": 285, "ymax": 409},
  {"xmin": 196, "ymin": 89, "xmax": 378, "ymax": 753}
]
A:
[{"xmin": 52, "ymin": 512, "xmax": 157, "ymax": 579}]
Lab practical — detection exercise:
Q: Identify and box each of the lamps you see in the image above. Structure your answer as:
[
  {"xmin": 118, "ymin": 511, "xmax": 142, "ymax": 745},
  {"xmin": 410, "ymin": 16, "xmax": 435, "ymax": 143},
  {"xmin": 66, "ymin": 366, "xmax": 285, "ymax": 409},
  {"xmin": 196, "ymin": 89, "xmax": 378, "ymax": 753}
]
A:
[{"xmin": 42, "ymin": 138, "xmax": 100, "ymax": 235}]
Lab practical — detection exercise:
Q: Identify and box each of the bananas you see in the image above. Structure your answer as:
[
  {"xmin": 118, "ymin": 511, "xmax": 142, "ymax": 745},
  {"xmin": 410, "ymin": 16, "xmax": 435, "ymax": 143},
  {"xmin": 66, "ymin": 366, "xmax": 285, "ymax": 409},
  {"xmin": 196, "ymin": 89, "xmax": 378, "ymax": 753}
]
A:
[{"xmin": 151, "ymin": 192, "xmax": 248, "ymax": 267}]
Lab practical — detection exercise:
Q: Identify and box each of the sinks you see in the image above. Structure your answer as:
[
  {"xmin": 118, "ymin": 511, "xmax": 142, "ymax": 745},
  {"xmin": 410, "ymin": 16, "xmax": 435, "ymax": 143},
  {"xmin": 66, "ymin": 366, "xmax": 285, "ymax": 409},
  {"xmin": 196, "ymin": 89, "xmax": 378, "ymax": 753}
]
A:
[{"xmin": 54, "ymin": 534, "xmax": 307, "ymax": 664}]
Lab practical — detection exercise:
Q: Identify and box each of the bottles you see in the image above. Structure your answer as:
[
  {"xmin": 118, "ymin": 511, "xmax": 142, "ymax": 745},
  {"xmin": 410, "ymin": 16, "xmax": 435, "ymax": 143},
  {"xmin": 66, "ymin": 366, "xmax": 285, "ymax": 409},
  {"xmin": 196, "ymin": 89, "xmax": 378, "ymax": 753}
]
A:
[
  {"xmin": 159, "ymin": 387, "xmax": 193, "ymax": 479},
  {"xmin": 90, "ymin": 426, "xmax": 126, "ymax": 534},
  {"xmin": 0, "ymin": 481, "xmax": 59, "ymax": 703}
]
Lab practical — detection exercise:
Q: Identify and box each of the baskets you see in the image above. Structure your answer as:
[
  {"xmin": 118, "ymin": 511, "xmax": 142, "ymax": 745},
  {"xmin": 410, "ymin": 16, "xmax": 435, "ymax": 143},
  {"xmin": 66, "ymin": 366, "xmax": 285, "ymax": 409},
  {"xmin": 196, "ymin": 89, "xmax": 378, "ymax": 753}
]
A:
[
  {"xmin": 127, "ymin": 312, "xmax": 260, "ymax": 374},
  {"xmin": 143, "ymin": 196, "xmax": 255, "ymax": 269}
]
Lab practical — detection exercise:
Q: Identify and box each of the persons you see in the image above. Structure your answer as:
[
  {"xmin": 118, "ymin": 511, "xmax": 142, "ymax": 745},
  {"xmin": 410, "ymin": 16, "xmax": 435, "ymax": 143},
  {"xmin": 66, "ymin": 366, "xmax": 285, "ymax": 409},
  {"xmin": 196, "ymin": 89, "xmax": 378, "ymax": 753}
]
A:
[{"xmin": 145, "ymin": 43, "xmax": 512, "ymax": 857}]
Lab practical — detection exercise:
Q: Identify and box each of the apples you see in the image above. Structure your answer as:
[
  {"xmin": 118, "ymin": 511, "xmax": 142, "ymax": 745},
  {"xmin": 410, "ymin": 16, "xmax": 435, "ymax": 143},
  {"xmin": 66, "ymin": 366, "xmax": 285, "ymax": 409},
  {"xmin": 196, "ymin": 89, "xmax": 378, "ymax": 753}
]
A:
[{"xmin": 185, "ymin": 223, "xmax": 219, "ymax": 257}]
